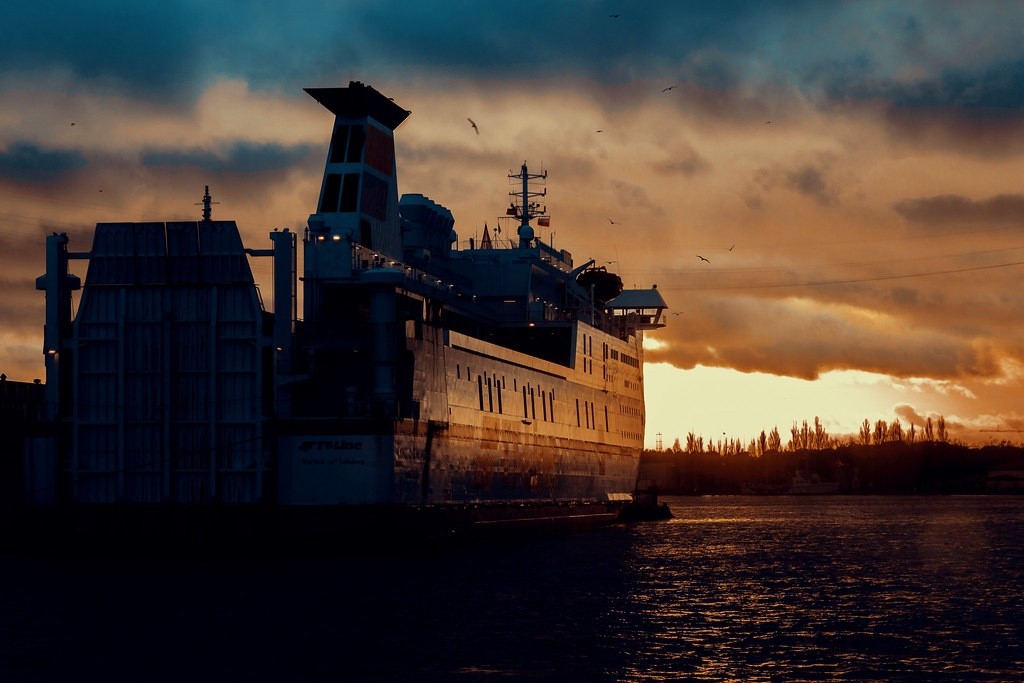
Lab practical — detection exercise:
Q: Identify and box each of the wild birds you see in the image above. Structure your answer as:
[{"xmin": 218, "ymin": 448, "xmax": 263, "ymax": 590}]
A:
[{"xmin": 465, "ymin": 13, "xmax": 736, "ymax": 316}]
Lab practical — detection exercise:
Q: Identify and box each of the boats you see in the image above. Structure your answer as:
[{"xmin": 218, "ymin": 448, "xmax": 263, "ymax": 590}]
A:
[
  {"xmin": 21, "ymin": 77, "xmax": 672, "ymax": 541},
  {"xmin": 618, "ymin": 480, "xmax": 676, "ymax": 522}
]
